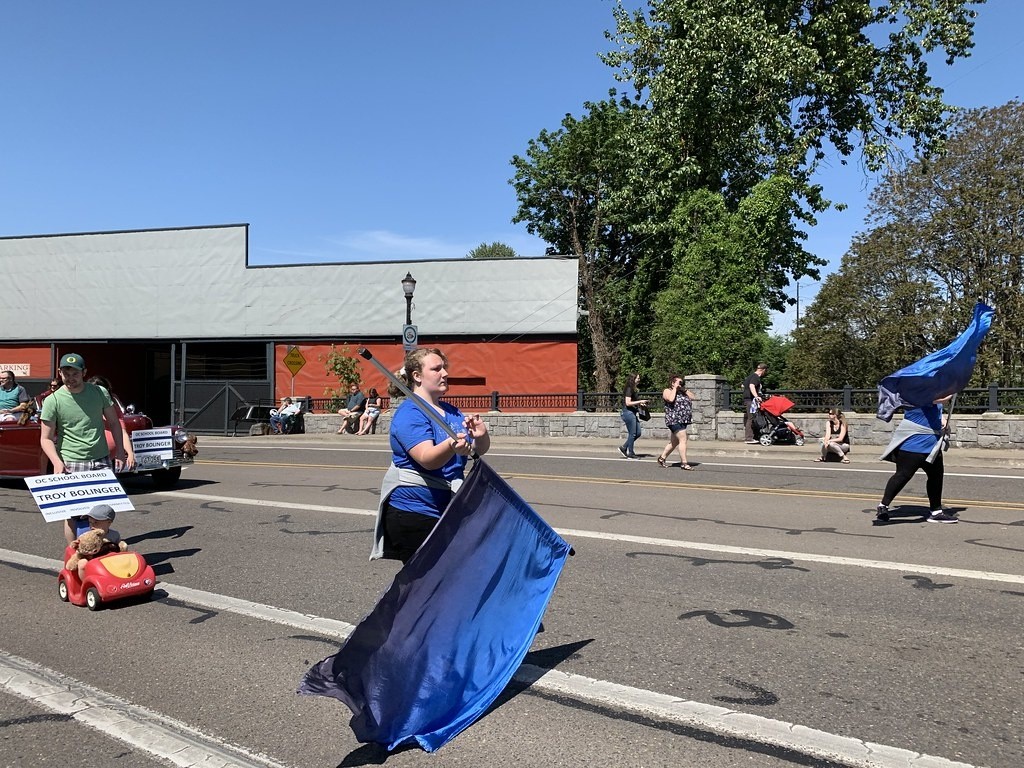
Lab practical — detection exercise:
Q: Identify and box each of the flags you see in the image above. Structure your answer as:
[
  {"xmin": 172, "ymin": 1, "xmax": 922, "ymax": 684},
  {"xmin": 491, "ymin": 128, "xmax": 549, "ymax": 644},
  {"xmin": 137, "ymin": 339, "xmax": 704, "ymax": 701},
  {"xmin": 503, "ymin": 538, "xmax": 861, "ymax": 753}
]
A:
[
  {"xmin": 297, "ymin": 457, "xmax": 572, "ymax": 753},
  {"xmin": 876, "ymin": 301, "xmax": 996, "ymax": 423}
]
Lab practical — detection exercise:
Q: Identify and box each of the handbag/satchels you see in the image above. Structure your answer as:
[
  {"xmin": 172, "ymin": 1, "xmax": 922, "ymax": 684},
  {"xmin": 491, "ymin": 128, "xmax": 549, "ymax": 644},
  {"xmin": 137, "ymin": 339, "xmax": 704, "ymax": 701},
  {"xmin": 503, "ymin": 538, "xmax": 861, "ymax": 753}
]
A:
[{"xmin": 639, "ymin": 404, "xmax": 651, "ymax": 422}]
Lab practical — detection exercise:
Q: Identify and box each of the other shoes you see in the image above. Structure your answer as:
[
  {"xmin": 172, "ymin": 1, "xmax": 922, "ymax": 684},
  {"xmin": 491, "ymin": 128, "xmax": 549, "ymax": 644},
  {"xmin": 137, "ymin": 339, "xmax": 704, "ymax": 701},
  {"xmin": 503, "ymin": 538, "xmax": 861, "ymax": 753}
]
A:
[
  {"xmin": 680, "ymin": 462, "xmax": 694, "ymax": 469},
  {"xmin": 815, "ymin": 457, "xmax": 826, "ymax": 462},
  {"xmin": 841, "ymin": 459, "xmax": 850, "ymax": 464},
  {"xmin": 658, "ymin": 455, "xmax": 668, "ymax": 468}
]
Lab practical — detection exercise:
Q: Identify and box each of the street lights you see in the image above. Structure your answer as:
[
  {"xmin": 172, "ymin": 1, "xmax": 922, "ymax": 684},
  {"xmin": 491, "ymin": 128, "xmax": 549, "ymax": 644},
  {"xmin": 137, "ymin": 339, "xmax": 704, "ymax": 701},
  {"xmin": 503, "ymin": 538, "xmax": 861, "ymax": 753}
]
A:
[{"xmin": 400, "ymin": 271, "xmax": 416, "ymax": 354}]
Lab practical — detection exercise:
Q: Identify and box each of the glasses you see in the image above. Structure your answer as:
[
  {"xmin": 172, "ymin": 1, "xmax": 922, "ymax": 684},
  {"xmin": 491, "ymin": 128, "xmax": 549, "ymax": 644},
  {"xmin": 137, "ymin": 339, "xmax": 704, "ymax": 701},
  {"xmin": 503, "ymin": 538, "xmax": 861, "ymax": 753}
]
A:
[{"xmin": 828, "ymin": 413, "xmax": 834, "ymax": 415}]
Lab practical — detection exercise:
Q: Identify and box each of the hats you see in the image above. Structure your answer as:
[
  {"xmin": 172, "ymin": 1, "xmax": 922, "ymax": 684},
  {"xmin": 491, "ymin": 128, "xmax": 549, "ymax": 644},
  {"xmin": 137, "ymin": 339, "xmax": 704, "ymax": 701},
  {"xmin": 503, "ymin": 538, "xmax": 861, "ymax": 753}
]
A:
[
  {"xmin": 80, "ymin": 504, "xmax": 116, "ymax": 521},
  {"xmin": 60, "ymin": 354, "xmax": 85, "ymax": 370},
  {"xmin": 758, "ymin": 363, "xmax": 768, "ymax": 369}
]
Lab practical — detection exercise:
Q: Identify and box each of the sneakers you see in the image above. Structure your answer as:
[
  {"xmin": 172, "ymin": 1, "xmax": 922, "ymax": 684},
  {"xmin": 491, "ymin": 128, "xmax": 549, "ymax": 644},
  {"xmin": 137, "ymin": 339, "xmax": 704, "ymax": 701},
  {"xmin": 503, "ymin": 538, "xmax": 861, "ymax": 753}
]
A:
[
  {"xmin": 618, "ymin": 446, "xmax": 641, "ymax": 461},
  {"xmin": 926, "ymin": 509, "xmax": 959, "ymax": 523},
  {"xmin": 877, "ymin": 505, "xmax": 890, "ymax": 521},
  {"xmin": 744, "ymin": 439, "xmax": 759, "ymax": 443}
]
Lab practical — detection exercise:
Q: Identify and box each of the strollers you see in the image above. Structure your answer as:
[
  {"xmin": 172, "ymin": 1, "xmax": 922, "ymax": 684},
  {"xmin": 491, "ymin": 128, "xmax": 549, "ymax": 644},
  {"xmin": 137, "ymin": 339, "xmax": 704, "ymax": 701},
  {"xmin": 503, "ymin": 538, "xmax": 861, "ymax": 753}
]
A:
[{"xmin": 754, "ymin": 396, "xmax": 806, "ymax": 446}]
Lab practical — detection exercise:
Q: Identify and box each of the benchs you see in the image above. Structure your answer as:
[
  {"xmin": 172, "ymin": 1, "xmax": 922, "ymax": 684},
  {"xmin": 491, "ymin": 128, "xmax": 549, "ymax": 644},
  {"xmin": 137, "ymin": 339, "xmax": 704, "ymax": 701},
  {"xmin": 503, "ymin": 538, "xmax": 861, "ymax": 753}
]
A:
[{"xmin": 229, "ymin": 406, "xmax": 304, "ymax": 437}]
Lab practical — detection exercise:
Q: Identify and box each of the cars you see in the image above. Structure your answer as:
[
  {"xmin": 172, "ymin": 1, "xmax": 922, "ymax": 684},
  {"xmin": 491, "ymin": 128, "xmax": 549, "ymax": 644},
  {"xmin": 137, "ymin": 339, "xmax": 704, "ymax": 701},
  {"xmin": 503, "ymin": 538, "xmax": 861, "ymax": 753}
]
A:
[
  {"xmin": 0, "ymin": 388, "xmax": 194, "ymax": 488},
  {"xmin": 57, "ymin": 543, "xmax": 157, "ymax": 612}
]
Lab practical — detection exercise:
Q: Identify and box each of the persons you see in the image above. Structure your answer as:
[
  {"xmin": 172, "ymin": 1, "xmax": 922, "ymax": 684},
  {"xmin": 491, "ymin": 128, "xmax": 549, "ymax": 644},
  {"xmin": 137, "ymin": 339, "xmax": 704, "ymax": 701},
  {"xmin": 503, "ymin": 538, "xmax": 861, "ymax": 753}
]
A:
[
  {"xmin": 617, "ymin": 372, "xmax": 649, "ymax": 460},
  {"xmin": 0, "ymin": 371, "xmax": 29, "ymax": 422},
  {"xmin": 369, "ymin": 347, "xmax": 490, "ymax": 565},
  {"xmin": 40, "ymin": 353, "xmax": 126, "ymax": 545},
  {"xmin": 355, "ymin": 388, "xmax": 382, "ymax": 435},
  {"xmin": 742, "ymin": 364, "xmax": 767, "ymax": 444},
  {"xmin": 270, "ymin": 397, "xmax": 300, "ymax": 435},
  {"xmin": 814, "ymin": 407, "xmax": 850, "ymax": 464},
  {"xmin": 81, "ymin": 505, "xmax": 128, "ymax": 553},
  {"xmin": 86, "ymin": 377, "xmax": 135, "ymax": 476},
  {"xmin": 658, "ymin": 375, "xmax": 694, "ymax": 470},
  {"xmin": 36, "ymin": 378, "xmax": 61, "ymax": 408},
  {"xmin": 337, "ymin": 385, "xmax": 366, "ymax": 434},
  {"xmin": 876, "ymin": 395, "xmax": 959, "ymax": 524}
]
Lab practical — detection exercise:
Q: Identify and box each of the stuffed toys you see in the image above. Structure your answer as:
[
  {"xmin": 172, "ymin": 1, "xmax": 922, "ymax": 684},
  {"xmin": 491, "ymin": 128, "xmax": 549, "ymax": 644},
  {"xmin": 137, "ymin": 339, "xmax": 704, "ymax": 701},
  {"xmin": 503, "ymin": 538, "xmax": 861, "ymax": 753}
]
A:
[{"xmin": 66, "ymin": 529, "xmax": 105, "ymax": 582}]
